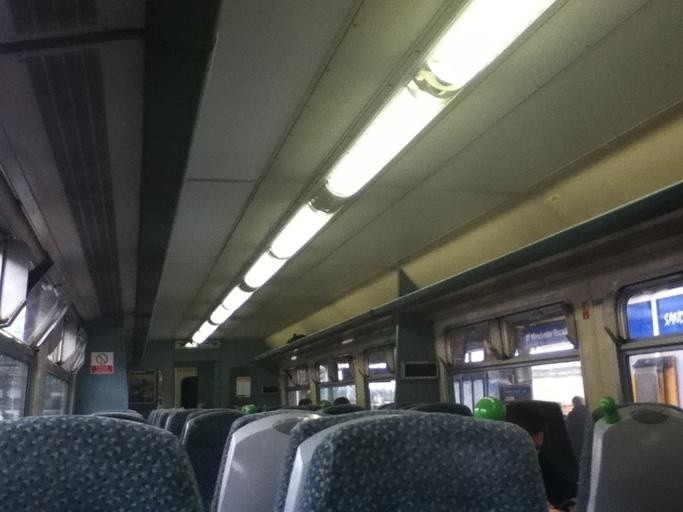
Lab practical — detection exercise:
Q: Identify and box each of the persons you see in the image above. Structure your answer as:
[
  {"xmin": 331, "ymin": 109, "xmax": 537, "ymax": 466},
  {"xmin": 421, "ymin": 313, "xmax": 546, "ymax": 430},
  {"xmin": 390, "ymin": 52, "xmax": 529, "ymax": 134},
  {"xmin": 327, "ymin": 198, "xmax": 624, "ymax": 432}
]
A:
[
  {"xmin": 505, "ymin": 408, "xmax": 566, "ymax": 512},
  {"xmin": 299, "ymin": 399, "xmax": 313, "ymax": 405},
  {"xmin": 333, "ymin": 397, "xmax": 349, "ymax": 405},
  {"xmin": 568, "ymin": 396, "xmax": 584, "ymax": 428}
]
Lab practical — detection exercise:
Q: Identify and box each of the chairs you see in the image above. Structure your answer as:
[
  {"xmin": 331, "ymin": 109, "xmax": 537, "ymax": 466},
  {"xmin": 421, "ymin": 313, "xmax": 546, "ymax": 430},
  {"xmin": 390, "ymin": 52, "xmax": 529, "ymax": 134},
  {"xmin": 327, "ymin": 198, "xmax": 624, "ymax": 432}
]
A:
[{"xmin": 0, "ymin": 401, "xmax": 682, "ymax": 511}]
